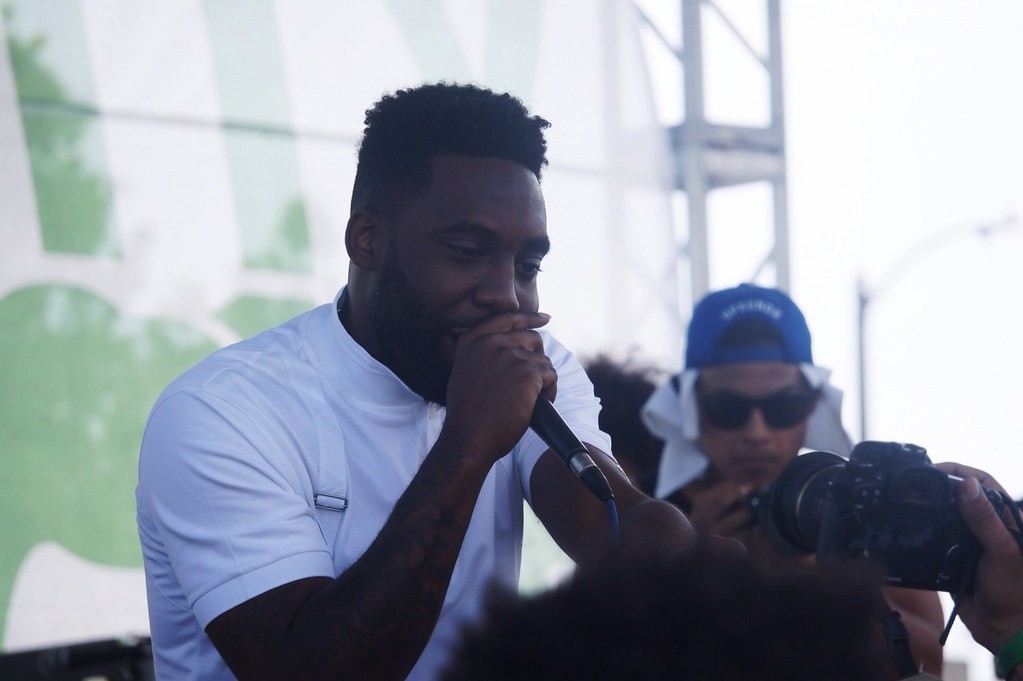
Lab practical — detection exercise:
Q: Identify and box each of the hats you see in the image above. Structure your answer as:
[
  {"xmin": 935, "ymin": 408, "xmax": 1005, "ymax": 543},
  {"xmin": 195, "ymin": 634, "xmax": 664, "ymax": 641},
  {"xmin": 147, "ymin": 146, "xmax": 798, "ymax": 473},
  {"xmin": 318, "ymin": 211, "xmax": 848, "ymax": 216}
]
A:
[{"xmin": 686, "ymin": 284, "xmax": 812, "ymax": 368}]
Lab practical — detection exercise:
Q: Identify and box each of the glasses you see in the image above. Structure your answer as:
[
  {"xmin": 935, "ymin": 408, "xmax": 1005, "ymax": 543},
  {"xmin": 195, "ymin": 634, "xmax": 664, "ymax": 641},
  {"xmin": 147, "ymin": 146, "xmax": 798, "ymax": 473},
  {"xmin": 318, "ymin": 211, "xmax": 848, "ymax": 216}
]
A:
[{"xmin": 699, "ymin": 387, "xmax": 815, "ymax": 429}]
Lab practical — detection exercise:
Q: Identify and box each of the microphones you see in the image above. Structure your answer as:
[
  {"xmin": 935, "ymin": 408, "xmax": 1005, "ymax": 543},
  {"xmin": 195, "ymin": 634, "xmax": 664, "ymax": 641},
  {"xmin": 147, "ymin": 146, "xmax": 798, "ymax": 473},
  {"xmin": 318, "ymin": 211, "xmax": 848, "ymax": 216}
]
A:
[{"xmin": 530, "ymin": 391, "xmax": 616, "ymax": 503}]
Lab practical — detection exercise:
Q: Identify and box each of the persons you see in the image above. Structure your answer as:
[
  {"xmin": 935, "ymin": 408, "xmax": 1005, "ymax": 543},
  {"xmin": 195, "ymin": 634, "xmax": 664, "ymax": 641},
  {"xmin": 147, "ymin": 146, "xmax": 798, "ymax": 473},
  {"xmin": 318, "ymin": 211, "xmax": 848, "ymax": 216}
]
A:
[
  {"xmin": 643, "ymin": 284, "xmax": 943, "ymax": 680},
  {"xmin": 137, "ymin": 81, "xmax": 745, "ymax": 680},
  {"xmin": 932, "ymin": 461, "xmax": 1023, "ymax": 680}
]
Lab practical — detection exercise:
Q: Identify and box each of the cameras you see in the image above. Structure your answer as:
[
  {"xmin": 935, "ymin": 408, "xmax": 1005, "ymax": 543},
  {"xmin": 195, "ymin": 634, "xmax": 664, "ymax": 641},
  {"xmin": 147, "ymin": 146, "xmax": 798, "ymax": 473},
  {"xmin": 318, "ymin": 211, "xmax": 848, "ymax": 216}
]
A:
[{"xmin": 771, "ymin": 439, "xmax": 1005, "ymax": 596}]
